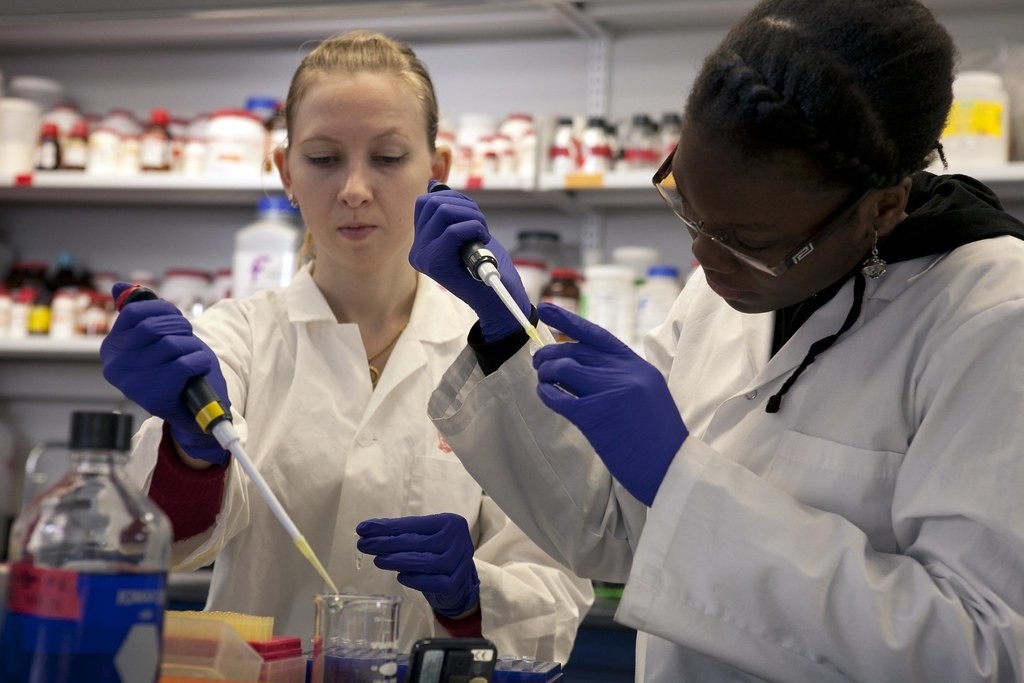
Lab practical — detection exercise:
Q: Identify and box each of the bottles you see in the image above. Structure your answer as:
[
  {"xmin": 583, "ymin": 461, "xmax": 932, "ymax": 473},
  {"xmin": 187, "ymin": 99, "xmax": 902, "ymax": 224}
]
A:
[
  {"xmin": 0, "ymin": 71, "xmax": 1012, "ymax": 338},
  {"xmin": 0, "ymin": 411, "xmax": 171, "ymax": 683}
]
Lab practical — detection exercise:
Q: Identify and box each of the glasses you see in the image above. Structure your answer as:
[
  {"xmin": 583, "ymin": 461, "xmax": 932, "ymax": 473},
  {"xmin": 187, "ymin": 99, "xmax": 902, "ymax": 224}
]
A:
[{"xmin": 651, "ymin": 142, "xmax": 872, "ymax": 277}]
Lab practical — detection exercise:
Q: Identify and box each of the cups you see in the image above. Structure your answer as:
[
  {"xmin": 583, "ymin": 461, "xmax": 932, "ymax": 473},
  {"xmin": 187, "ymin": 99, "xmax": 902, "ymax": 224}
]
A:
[{"xmin": 311, "ymin": 594, "xmax": 402, "ymax": 683}]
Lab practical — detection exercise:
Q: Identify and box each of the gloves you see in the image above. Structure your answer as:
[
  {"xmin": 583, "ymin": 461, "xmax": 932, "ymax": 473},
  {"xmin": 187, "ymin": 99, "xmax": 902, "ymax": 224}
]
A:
[
  {"xmin": 356, "ymin": 513, "xmax": 481, "ymax": 618},
  {"xmin": 532, "ymin": 303, "xmax": 689, "ymax": 508},
  {"xmin": 100, "ymin": 282, "xmax": 233, "ymax": 467},
  {"xmin": 409, "ymin": 180, "xmax": 534, "ymax": 342}
]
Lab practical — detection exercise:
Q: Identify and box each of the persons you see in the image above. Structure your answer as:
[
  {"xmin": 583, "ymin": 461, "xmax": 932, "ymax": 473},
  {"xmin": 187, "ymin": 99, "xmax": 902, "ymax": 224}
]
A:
[
  {"xmin": 407, "ymin": 0, "xmax": 1024, "ymax": 683},
  {"xmin": 99, "ymin": 33, "xmax": 595, "ymax": 670}
]
